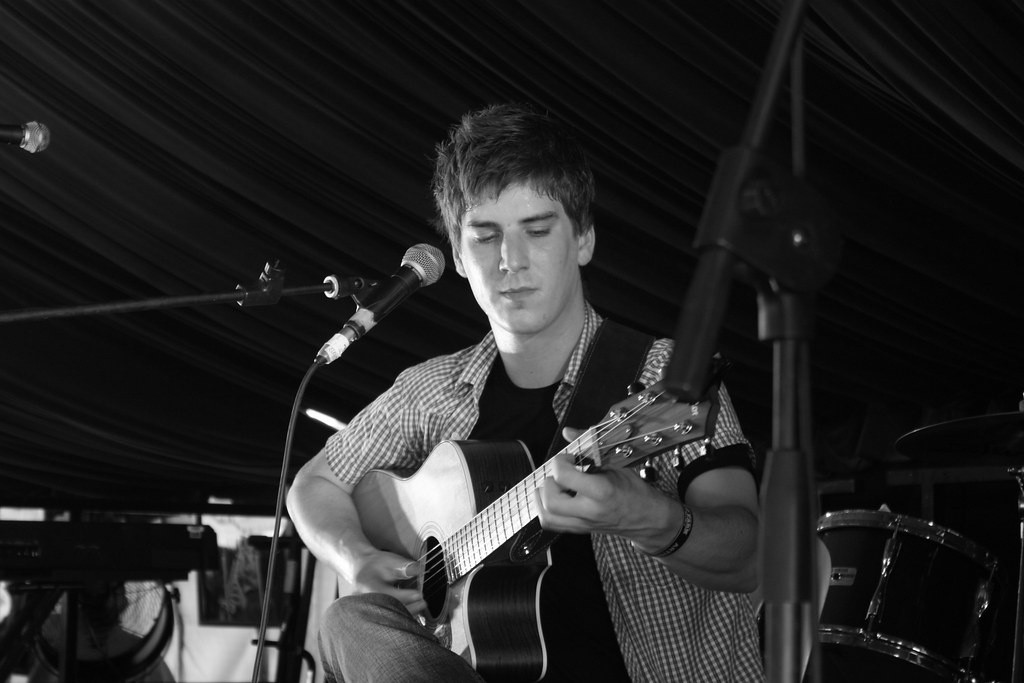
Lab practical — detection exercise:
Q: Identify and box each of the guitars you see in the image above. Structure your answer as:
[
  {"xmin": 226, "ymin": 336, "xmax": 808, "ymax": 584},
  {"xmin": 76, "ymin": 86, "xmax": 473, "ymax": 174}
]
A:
[{"xmin": 302, "ymin": 366, "xmax": 720, "ymax": 683}]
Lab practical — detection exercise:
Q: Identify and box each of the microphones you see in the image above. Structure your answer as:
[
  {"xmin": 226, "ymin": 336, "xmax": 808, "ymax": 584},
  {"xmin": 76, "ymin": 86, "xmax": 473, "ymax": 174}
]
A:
[
  {"xmin": 313, "ymin": 244, "xmax": 445, "ymax": 367},
  {"xmin": 0, "ymin": 120, "xmax": 50, "ymax": 153}
]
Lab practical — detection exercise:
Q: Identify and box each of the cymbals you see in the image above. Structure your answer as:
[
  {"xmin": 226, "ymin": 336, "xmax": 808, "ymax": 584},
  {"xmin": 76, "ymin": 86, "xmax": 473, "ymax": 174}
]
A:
[{"xmin": 894, "ymin": 409, "xmax": 1024, "ymax": 468}]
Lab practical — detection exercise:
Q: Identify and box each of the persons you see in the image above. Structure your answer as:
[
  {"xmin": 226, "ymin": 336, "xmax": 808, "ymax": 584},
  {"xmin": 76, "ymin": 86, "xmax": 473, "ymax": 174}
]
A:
[{"xmin": 285, "ymin": 105, "xmax": 769, "ymax": 683}]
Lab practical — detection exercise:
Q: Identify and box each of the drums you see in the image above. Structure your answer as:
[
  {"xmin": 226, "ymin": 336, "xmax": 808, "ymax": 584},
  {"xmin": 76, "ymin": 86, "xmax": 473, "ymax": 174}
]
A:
[{"xmin": 809, "ymin": 509, "xmax": 1004, "ymax": 683}]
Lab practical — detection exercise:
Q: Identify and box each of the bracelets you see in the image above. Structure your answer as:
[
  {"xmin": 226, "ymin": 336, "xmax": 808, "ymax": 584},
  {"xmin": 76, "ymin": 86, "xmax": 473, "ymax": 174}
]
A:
[{"xmin": 631, "ymin": 499, "xmax": 696, "ymax": 559}]
